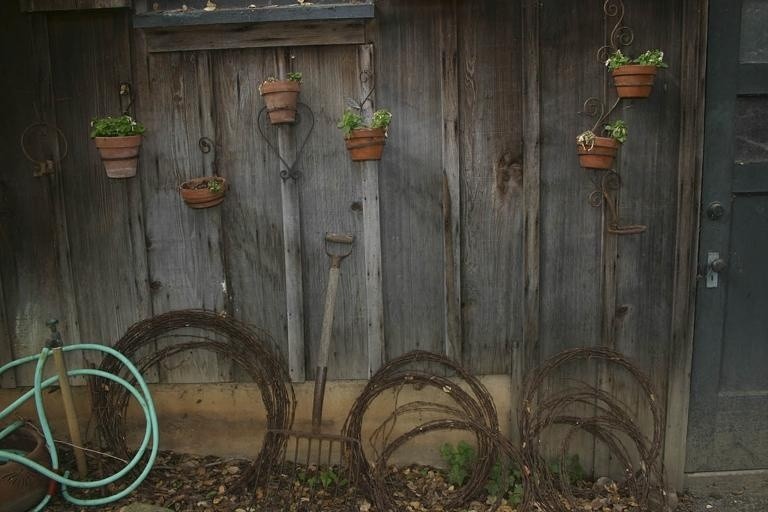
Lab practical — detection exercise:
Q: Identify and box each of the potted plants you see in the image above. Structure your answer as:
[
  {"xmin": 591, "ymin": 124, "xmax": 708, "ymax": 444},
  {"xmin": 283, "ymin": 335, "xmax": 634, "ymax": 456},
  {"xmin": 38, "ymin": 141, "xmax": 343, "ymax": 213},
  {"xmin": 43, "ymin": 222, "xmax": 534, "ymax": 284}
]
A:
[
  {"xmin": 336, "ymin": 108, "xmax": 394, "ymax": 160},
  {"xmin": 257, "ymin": 72, "xmax": 304, "ymax": 124},
  {"xmin": 575, "ymin": 121, "xmax": 628, "ymax": 170},
  {"xmin": 176, "ymin": 175, "xmax": 227, "ymax": 209},
  {"xmin": 89, "ymin": 113, "xmax": 146, "ymax": 178},
  {"xmin": 605, "ymin": 47, "xmax": 668, "ymax": 98}
]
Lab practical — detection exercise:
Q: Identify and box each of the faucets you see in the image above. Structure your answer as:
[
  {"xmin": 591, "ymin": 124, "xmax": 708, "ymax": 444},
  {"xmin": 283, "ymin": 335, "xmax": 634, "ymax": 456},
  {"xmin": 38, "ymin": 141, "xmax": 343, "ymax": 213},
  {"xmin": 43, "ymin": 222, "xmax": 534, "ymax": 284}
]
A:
[{"xmin": 40, "ymin": 318, "xmax": 64, "ymax": 350}]
